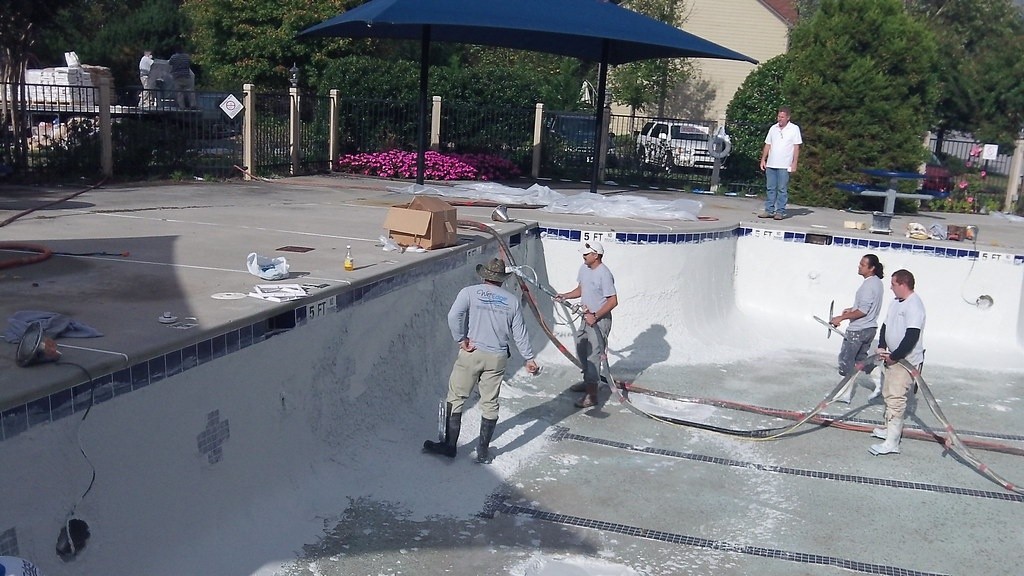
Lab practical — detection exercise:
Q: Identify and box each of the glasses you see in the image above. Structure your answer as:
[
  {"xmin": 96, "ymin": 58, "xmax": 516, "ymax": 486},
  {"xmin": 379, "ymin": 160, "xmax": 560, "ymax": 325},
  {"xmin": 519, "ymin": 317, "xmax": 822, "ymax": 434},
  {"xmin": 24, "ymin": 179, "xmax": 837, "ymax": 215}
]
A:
[{"xmin": 585, "ymin": 243, "xmax": 598, "ymax": 252}]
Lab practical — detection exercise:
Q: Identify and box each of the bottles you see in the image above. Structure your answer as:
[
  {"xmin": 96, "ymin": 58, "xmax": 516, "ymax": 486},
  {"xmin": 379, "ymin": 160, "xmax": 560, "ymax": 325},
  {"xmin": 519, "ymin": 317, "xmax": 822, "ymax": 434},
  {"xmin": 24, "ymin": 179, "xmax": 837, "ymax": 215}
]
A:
[{"xmin": 344, "ymin": 246, "xmax": 354, "ymax": 271}]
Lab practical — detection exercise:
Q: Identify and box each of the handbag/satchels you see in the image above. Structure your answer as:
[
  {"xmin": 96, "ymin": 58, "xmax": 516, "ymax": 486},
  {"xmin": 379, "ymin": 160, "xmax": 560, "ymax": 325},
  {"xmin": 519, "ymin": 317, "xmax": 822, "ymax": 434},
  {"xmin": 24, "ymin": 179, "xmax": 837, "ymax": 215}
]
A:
[{"xmin": 246, "ymin": 252, "xmax": 290, "ymax": 281}]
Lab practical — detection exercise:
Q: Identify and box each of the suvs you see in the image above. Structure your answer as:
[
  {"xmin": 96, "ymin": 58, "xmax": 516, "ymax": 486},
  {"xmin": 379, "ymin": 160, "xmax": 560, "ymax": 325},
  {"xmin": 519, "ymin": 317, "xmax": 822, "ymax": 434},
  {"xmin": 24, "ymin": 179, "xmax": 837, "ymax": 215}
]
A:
[
  {"xmin": 546, "ymin": 114, "xmax": 622, "ymax": 171},
  {"xmin": 637, "ymin": 121, "xmax": 726, "ymax": 173}
]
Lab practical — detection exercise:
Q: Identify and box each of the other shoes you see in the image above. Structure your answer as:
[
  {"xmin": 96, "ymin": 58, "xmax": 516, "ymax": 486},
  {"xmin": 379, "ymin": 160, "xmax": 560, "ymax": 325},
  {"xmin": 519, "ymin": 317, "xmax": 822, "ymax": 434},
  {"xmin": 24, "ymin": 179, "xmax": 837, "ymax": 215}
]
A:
[
  {"xmin": 758, "ymin": 211, "xmax": 773, "ymax": 218},
  {"xmin": 773, "ymin": 213, "xmax": 785, "ymax": 220}
]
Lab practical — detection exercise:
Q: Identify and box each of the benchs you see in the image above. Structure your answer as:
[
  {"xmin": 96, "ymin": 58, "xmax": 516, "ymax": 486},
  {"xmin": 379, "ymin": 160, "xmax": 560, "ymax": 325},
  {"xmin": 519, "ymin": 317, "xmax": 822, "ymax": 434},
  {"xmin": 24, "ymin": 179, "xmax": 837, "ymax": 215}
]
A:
[
  {"xmin": 837, "ymin": 182, "xmax": 951, "ymax": 215},
  {"xmin": 860, "ymin": 167, "xmax": 930, "ymax": 217}
]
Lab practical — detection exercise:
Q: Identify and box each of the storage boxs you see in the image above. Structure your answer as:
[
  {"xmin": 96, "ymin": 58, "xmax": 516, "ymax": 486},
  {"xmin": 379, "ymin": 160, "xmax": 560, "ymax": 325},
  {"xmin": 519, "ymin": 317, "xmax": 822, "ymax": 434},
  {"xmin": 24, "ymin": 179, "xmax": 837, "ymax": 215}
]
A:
[{"xmin": 383, "ymin": 193, "xmax": 457, "ymax": 250}]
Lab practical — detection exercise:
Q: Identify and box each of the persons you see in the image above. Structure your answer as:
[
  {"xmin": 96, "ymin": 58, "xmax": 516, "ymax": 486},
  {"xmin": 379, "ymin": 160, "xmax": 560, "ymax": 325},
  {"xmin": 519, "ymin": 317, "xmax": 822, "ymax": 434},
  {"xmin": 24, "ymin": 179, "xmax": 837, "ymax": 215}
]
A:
[
  {"xmin": 824, "ymin": 254, "xmax": 884, "ymax": 402},
  {"xmin": 871, "ymin": 269, "xmax": 926, "ymax": 453},
  {"xmin": 138, "ymin": 50, "xmax": 154, "ymax": 107},
  {"xmin": 758, "ymin": 108, "xmax": 802, "ymax": 220},
  {"xmin": 553, "ymin": 242, "xmax": 619, "ymax": 407},
  {"xmin": 169, "ymin": 44, "xmax": 200, "ymax": 110},
  {"xmin": 424, "ymin": 258, "xmax": 537, "ymax": 463}
]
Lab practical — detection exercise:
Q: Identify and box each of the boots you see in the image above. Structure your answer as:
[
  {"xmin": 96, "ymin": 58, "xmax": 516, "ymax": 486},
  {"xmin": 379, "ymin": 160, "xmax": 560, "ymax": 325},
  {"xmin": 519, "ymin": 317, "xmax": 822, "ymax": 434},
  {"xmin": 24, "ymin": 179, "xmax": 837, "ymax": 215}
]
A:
[
  {"xmin": 570, "ymin": 380, "xmax": 585, "ymax": 392},
  {"xmin": 824, "ymin": 376, "xmax": 856, "ymax": 404},
  {"xmin": 872, "ymin": 415, "xmax": 903, "ymax": 440},
  {"xmin": 870, "ymin": 419, "xmax": 903, "ymax": 454},
  {"xmin": 477, "ymin": 415, "xmax": 499, "ymax": 463},
  {"xmin": 868, "ymin": 366, "xmax": 885, "ymax": 400},
  {"xmin": 422, "ymin": 412, "xmax": 462, "ymax": 458},
  {"xmin": 574, "ymin": 383, "xmax": 599, "ymax": 408}
]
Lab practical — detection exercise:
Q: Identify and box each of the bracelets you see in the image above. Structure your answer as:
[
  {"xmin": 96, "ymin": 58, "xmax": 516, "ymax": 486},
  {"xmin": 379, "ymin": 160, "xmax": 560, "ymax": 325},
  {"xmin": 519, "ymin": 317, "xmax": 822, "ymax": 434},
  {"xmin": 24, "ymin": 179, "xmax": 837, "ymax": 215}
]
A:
[
  {"xmin": 525, "ymin": 357, "xmax": 533, "ymax": 361},
  {"xmin": 593, "ymin": 313, "xmax": 599, "ymax": 320}
]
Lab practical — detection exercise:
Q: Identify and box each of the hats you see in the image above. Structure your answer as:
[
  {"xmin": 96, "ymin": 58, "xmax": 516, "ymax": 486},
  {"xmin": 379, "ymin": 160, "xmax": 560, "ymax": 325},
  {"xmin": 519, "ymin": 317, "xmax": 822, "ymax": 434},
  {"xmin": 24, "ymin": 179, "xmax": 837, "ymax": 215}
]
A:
[
  {"xmin": 475, "ymin": 258, "xmax": 514, "ymax": 282},
  {"xmin": 578, "ymin": 241, "xmax": 604, "ymax": 254}
]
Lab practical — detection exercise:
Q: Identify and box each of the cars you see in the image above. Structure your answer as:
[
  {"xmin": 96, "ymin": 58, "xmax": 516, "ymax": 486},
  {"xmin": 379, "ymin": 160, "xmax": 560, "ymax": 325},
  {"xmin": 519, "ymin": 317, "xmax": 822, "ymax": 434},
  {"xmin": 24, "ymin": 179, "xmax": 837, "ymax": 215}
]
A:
[{"xmin": 926, "ymin": 151, "xmax": 956, "ymax": 193}]
